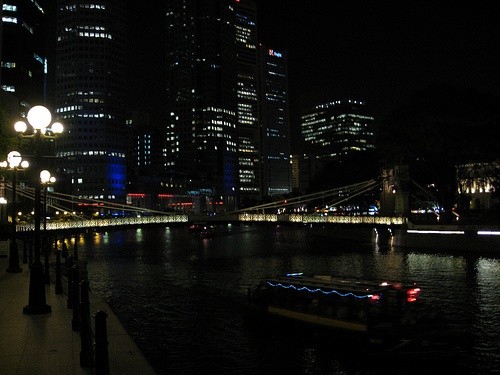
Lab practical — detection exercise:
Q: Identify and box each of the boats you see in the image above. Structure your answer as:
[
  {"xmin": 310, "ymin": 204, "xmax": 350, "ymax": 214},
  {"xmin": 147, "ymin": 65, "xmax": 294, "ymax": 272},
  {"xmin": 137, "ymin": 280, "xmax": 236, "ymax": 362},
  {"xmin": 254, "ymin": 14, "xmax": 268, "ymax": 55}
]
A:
[{"xmin": 248, "ymin": 271, "xmax": 443, "ymax": 355}]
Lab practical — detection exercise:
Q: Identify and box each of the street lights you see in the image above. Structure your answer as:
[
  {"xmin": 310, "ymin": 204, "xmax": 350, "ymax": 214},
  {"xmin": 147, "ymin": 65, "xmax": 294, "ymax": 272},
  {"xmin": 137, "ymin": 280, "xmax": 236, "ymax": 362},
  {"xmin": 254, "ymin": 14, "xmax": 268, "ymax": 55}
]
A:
[
  {"xmin": 13, "ymin": 105, "xmax": 65, "ymax": 317},
  {"xmin": 0, "ymin": 151, "xmax": 30, "ymax": 275}
]
